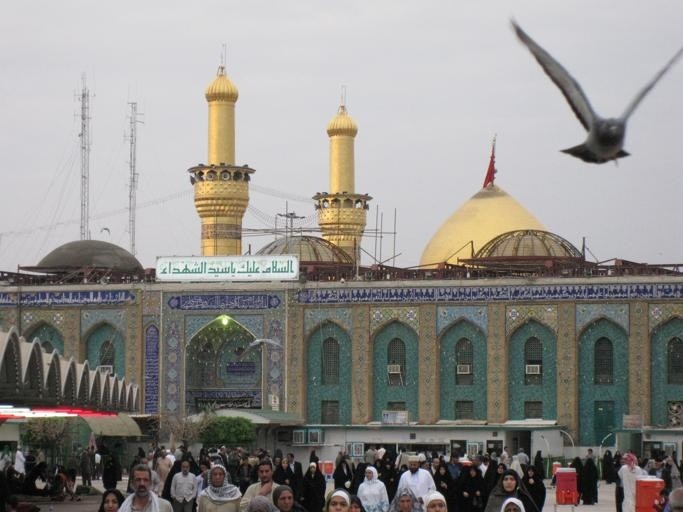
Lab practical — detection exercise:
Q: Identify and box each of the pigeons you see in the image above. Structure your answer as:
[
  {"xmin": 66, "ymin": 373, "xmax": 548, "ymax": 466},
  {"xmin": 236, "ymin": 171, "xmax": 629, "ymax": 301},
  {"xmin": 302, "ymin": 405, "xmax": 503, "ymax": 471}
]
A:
[{"xmin": 507, "ymin": 16, "xmax": 683, "ymax": 164}]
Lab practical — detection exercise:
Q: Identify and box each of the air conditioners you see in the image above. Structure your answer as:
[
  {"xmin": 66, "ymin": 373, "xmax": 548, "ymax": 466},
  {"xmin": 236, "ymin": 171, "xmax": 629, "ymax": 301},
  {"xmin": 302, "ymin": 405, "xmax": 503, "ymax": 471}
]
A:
[
  {"xmin": 292, "ymin": 429, "xmax": 307, "ymax": 444},
  {"xmin": 308, "ymin": 429, "xmax": 324, "ymax": 443},
  {"xmin": 524, "ymin": 364, "xmax": 541, "ymax": 374},
  {"xmin": 456, "ymin": 365, "xmax": 470, "ymax": 375},
  {"xmin": 386, "ymin": 364, "xmax": 401, "ymax": 375},
  {"xmin": 98, "ymin": 365, "xmax": 113, "ymax": 374}
]
